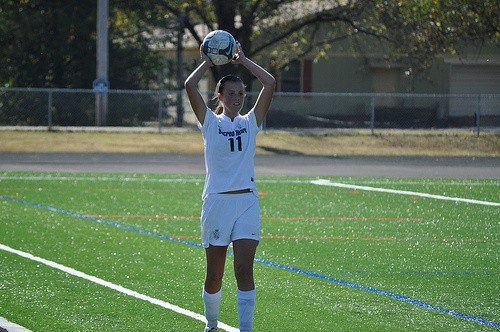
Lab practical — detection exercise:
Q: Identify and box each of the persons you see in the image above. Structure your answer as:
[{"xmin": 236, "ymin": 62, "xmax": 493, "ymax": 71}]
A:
[{"xmin": 184, "ymin": 40, "xmax": 277, "ymax": 332}]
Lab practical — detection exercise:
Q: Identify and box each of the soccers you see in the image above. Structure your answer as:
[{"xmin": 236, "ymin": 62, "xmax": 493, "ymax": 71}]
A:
[{"xmin": 201, "ymin": 30, "xmax": 237, "ymax": 65}]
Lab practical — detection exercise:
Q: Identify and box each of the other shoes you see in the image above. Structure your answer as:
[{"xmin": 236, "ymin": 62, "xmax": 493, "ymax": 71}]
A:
[{"xmin": 203, "ymin": 323, "xmax": 217, "ymax": 332}]
